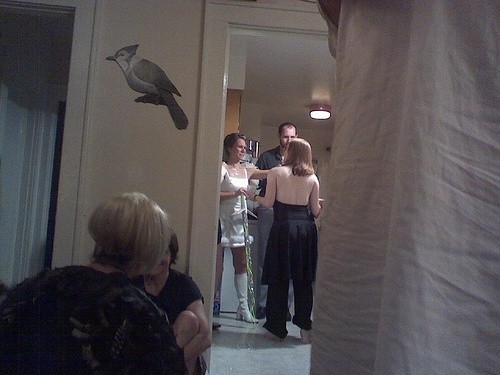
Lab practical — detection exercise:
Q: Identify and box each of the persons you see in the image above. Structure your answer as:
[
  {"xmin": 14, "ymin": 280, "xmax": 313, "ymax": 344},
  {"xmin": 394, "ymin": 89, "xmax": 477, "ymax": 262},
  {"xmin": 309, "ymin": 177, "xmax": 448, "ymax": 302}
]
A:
[
  {"xmin": 244, "ymin": 138, "xmax": 321, "ymax": 344},
  {"xmin": 135, "ymin": 228, "xmax": 213, "ymax": 375},
  {"xmin": 318, "ymin": 0, "xmax": 500, "ymax": 374},
  {"xmin": 255, "ymin": 123, "xmax": 302, "ymax": 322},
  {"xmin": 0, "ymin": 191, "xmax": 182, "ymax": 375},
  {"xmin": 211, "ymin": 132, "xmax": 283, "ymax": 329}
]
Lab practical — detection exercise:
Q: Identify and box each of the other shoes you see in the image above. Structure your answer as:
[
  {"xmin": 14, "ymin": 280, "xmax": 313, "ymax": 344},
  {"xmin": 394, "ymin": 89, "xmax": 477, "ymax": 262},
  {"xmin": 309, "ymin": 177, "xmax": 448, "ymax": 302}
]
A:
[
  {"xmin": 287, "ymin": 308, "xmax": 291, "ymax": 321},
  {"xmin": 256, "ymin": 308, "xmax": 264, "ymax": 320}
]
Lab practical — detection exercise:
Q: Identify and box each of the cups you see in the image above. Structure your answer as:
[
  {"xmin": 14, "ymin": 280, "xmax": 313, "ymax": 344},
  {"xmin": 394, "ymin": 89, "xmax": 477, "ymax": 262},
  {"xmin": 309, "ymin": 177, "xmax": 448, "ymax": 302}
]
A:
[{"xmin": 247, "ymin": 185, "xmax": 257, "ymax": 194}]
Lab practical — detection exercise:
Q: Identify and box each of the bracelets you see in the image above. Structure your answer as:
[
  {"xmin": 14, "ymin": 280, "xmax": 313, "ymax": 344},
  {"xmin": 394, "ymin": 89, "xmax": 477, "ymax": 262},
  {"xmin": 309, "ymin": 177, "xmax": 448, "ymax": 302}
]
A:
[{"xmin": 253, "ymin": 194, "xmax": 258, "ymax": 200}]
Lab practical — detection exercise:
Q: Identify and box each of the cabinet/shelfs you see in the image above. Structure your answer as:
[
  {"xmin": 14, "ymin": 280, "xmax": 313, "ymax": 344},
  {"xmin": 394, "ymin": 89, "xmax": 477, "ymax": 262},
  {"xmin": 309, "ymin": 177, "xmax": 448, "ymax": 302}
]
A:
[{"xmin": 225, "ymin": 91, "xmax": 241, "ymax": 137}]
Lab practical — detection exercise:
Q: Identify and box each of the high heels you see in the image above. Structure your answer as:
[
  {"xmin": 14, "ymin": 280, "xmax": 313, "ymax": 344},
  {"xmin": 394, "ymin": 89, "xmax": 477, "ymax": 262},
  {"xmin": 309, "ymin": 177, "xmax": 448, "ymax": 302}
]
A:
[{"xmin": 234, "ymin": 272, "xmax": 260, "ymax": 323}]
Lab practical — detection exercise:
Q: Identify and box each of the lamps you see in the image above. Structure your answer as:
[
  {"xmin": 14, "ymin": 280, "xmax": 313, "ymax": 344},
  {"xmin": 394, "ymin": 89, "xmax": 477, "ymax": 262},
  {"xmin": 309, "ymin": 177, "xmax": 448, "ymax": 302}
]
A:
[{"xmin": 310, "ymin": 105, "xmax": 331, "ymax": 119}]
